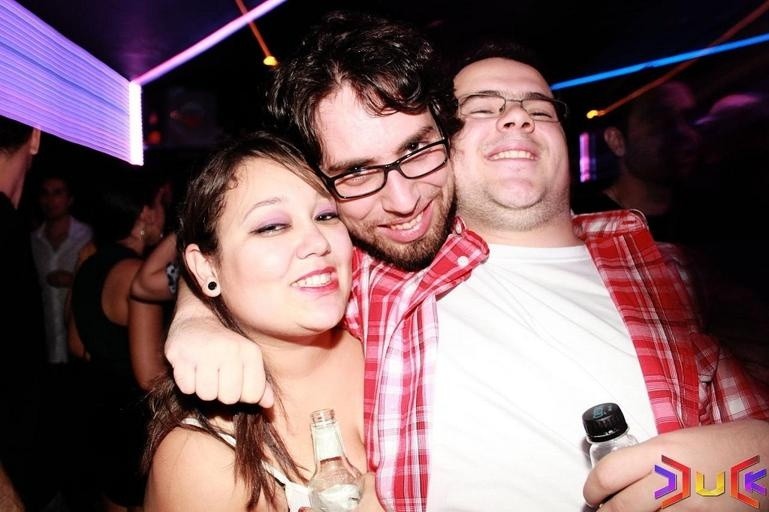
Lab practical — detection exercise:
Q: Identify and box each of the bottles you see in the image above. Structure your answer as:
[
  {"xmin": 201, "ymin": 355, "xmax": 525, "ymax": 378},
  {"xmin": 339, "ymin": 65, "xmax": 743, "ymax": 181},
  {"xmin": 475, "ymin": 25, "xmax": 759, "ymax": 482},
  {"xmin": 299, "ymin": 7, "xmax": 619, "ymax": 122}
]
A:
[
  {"xmin": 309, "ymin": 409, "xmax": 363, "ymax": 510},
  {"xmin": 581, "ymin": 401, "xmax": 637, "ymax": 473}
]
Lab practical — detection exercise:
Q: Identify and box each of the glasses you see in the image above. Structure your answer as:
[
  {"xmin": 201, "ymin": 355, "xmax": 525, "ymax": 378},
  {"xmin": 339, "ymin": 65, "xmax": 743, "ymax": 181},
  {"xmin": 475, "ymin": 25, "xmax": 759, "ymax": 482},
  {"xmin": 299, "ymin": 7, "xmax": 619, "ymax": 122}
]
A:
[
  {"xmin": 459, "ymin": 94, "xmax": 569, "ymax": 122},
  {"xmin": 315, "ymin": 107, "xmax": 450, "ymax": 199}
]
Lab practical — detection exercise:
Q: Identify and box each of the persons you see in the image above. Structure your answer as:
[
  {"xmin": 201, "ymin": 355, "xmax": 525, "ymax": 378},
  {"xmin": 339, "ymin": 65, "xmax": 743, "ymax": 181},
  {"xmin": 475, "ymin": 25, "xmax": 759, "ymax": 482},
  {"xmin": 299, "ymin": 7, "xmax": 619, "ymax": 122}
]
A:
[
  {"xmin": 587, "ymin": 67, "xmax": 706, "ymax": 261},
  {"xmin": 142, "ymin": 133, "xmax": 394, "ymax": 512},
  {"xmin": 261, "ymin": 4, "xmax": 453, "ymax": 278},
  {"xmin": 163, "ymin": 46, "xmax": 769, "ymax": 512}
]
